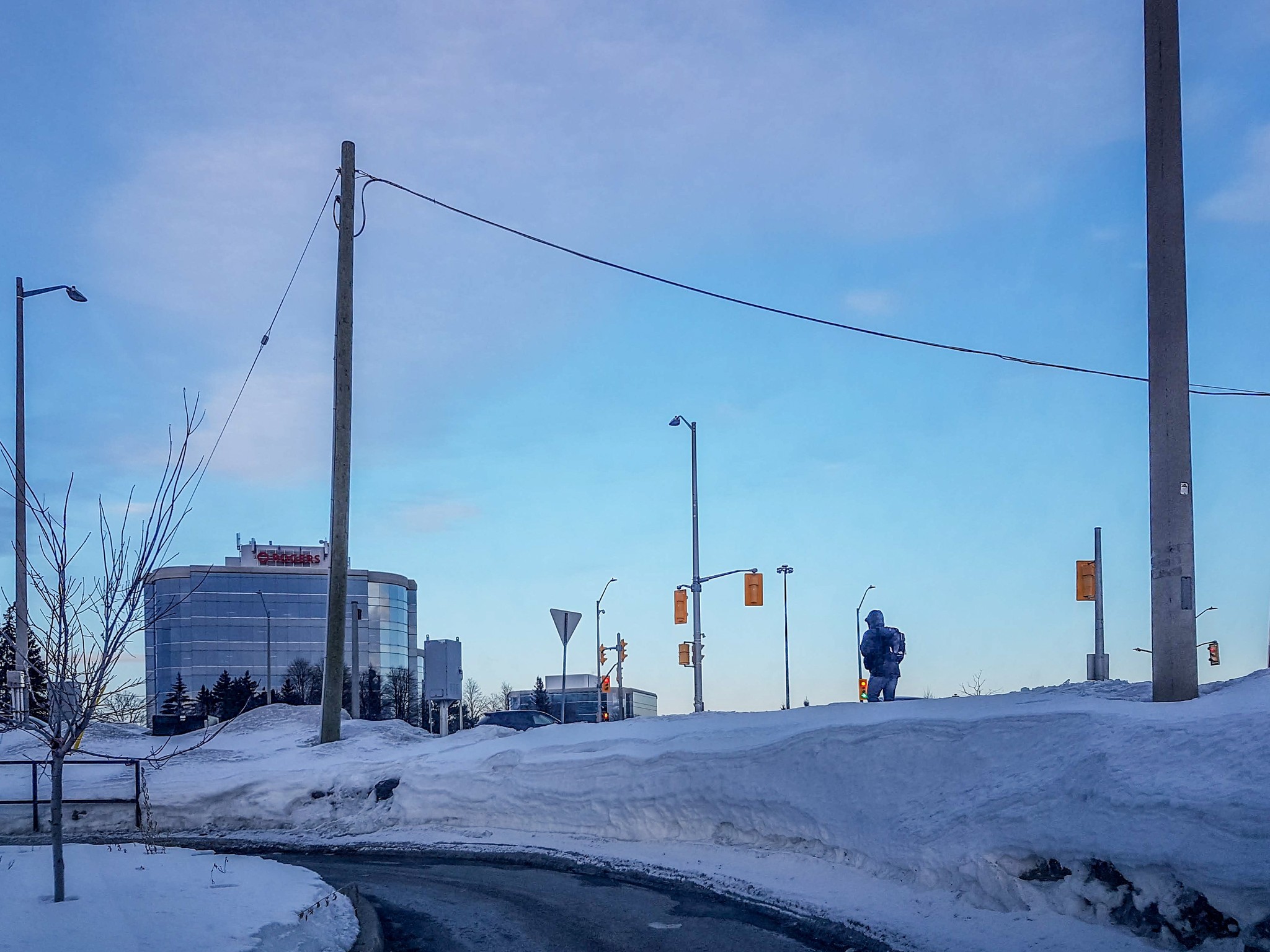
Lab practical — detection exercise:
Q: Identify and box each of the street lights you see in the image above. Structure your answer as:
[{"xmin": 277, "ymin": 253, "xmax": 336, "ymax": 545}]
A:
[
  {"xmin": 596, "ymin": 578, "xmax": 617, "ymax": 722},
  {"xmin": 776, "ymin": 564, "xmax": 794, "ymax": 709},
  {"xmin": 855, "ymin": 585, "xmax": 876, "ymax": 706},
  {"xmin": 14, "ymin": 276, "xmax": 89, "ymax": 713},
  {"xmin": 255, "ymin": 590, "xmax": 272, "ymax": 705},
  {"xmin": 667, "ymin": 413, "xmax": 704, "ymax": 715}
]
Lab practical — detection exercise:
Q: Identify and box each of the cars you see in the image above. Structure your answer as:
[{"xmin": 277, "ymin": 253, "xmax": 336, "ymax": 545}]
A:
[{"xmin": 475, "ymin": 709, "xmax": 562, "ymax": 733}]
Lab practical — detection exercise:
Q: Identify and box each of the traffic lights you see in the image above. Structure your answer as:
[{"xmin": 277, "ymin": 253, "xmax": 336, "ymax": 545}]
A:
[
  {"xmin": 602, "ymin": 675, "xmax": 611, "ymax": 692},
  {"xmin": 600, "ymin": 643, "xmax": 607, "ymax": 665},
  {"xmin": 603, "ymin": 712, "xmax": 609, "ymax": 719},
  {"xmin": 859, "ymin": 679, "xmax": 868, "ymax": 701},
  {"xmin": 1208, "ymin": 642, "xmax": 1221, "ymax": 666},
  {"xmin": 621, "ymin": 638, "xmax": 628, "ymax": 663}
]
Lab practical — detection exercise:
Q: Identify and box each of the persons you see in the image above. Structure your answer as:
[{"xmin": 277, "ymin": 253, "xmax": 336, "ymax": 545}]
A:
[{"xmin": 860, "ymin": 610, "xmax": 901, "ymax": 702}]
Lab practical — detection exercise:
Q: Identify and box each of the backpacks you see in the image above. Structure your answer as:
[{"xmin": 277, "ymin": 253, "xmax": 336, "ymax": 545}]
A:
[{"xmin": 874, "ymin": 627, "xmax": 905, "ymax": 661}]
